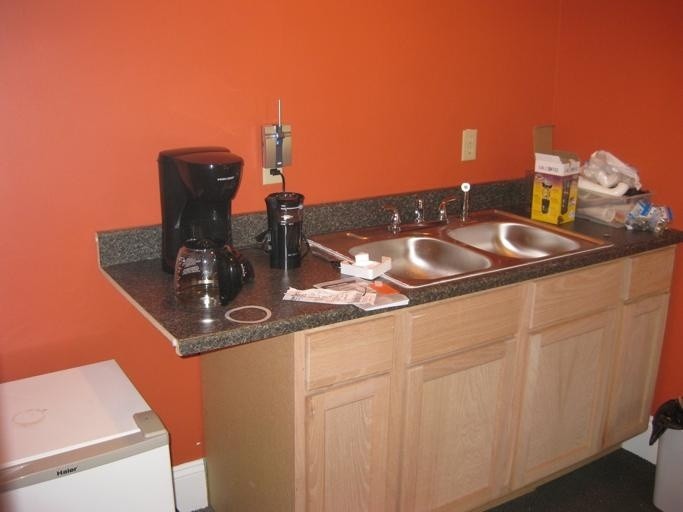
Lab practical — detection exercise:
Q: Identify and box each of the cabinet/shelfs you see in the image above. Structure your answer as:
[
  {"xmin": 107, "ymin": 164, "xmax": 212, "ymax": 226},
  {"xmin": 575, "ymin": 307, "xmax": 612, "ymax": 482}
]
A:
[
  {"xmin": 403, "ymin": 276, "xmax": 534, "ymax": 510},
  {"xmin": 505, "ymin": 255, "xmax": 632, "ymax": 497},
  {"xmin": 601, "ymin": 242, "xmax": 680, "ymax": 454},
  {"xmin": 203, "ymin": 312, "xmax": 401, "ymax": 511}
]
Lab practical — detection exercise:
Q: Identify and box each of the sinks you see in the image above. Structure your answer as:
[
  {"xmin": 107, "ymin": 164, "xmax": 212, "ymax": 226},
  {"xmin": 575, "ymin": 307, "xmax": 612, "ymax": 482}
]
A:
[
  {"xmin": 306, "ymin": 224, "xmax": 502, "ymax": 290},
  {"xmin": 434, "ymin": 208, "xmax": 615, "ymax": 260}
]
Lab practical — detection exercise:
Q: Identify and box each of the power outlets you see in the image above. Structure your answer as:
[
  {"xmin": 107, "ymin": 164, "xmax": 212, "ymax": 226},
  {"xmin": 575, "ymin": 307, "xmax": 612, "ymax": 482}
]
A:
[{"xmin": 262, "ymin": 166, "xmax": 283, "ymax": 186}]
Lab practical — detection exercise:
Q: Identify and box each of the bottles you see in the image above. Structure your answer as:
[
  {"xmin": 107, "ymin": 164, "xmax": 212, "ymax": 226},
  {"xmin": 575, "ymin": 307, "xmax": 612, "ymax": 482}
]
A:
[
  {"xmin": 541, "ymin": 181, "xmax": 551, "ymax": 214},
  {"xmin": 175, "ymin": 237, "xmax": 238, "ymax": 302},
  {"xmin": 561, "ymin": 178, "xmax": 570, "ymax": 214}
]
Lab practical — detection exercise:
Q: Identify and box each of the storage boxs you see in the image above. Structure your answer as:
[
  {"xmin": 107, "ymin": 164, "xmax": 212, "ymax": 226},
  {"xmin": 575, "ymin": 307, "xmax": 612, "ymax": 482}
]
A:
[
  {"xmin": 575, "ymin": 182, "xmax": 653, "ymax": 229},
  {"xmin": 531, "ymin": 121, "xmax": 581, "ymax": 226}
]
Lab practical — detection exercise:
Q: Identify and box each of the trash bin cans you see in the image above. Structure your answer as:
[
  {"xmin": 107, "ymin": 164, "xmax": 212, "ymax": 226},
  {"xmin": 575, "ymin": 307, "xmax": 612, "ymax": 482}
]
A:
[{"xmin": 652, "ymin": 397, "xmax": 683, "ymax": 512}]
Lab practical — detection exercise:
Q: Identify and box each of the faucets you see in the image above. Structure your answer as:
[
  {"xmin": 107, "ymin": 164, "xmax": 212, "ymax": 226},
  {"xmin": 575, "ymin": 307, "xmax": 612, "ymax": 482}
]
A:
[{"xmin": 413, "ymin": 198, "xmax": 425, "ymax": 224}]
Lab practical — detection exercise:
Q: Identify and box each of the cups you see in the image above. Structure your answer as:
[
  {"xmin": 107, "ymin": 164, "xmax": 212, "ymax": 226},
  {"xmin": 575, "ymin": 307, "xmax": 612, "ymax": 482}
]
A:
[{"xmin": 264, "ymin": 192, "xmax": 306, "ymax": 269}]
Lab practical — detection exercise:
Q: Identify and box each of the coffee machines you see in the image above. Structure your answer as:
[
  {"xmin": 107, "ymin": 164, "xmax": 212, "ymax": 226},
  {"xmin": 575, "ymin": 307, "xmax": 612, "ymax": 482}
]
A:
[{"xmin": 156, "ymin": 146, "xmax": 253, "ymax": 282}]
